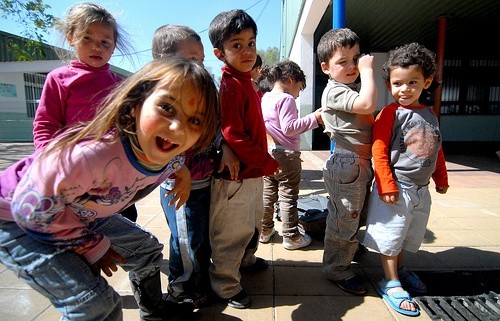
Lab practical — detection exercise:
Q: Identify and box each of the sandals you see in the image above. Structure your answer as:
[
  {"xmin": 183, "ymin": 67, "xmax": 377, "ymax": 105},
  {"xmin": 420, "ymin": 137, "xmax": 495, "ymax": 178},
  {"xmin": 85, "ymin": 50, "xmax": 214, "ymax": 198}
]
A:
[
  {"xmin": 397, "ymin": 265, "xmax": 427, "ymax": 293},
  {"xmin": 376, "ymin": 277, "xmax": 420, "ymax": 316},
  {"xmin": 239, "ymin": 257, "xmax": 268, "ymax": 273}
]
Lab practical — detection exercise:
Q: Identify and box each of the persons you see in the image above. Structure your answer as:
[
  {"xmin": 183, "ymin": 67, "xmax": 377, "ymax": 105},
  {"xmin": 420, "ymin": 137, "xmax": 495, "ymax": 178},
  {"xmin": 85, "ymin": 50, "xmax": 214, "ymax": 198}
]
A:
[
  {"xmin": 207, "ymin": 6, "xmax": 284, "ymax": 311},
  {"xmin": 151, "ymin": 22, "xmax": 218, "ymax": 308},
  {"xmin": 362, "ymin": 42, "xmax": 449, "ymax": 317},
  {"xmin": 0, "ymin": 57, "xmax": 219, "ymax": 321},
  {"xmin": 248, "ymin": 53, "xmax": 274, "ymax": 103},
  {"xmin": 316, "ymin": 27, "xmax": 379, "ymax": 296},
  {"xmin": 32, "ymin": 2, "xmax": 139, "ymax": 224},
  {"xmin": 256, "ymin": 59, "xmax": 325, "ymax": 250}
]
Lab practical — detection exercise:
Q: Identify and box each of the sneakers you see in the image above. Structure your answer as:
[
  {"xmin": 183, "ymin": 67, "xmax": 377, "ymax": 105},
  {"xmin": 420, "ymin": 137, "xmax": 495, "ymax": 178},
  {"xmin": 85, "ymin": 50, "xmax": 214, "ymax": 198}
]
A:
[
  {"xmin": 283, "ymin": 233, "xmax": 312, "ymax": 249},
  {"xmin": 259, "ymin": 228, "xmax": 276, "ymax": 242}
]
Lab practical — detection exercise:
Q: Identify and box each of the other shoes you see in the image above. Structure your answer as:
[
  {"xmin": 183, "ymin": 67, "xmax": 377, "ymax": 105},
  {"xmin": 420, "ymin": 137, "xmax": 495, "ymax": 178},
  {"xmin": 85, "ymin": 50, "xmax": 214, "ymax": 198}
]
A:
[
  {"xmin": 223, "ymin": 289, "xmax": 252, "ymax": 308},
  {"xmin": 335, "ymin": 274, "xmax": 369, "ymax": 294},
  {"xmin": 352, "ymin": 243, "xmax": 375, "ymax": 265},
  {"xmin": 168, "ymin": 287, "xmax": 208, "ymax": 307}
]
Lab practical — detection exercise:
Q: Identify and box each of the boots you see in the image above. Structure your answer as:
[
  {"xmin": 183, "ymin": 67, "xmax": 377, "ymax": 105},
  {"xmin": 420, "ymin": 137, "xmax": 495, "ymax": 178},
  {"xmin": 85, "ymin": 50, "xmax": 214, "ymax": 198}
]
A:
[{"xmin": 131, "ymin": 270, "xmax": 196, "ymax": 321}]
